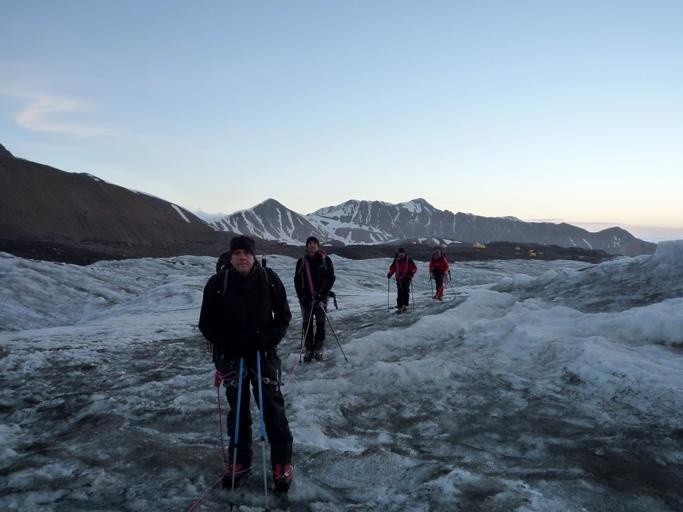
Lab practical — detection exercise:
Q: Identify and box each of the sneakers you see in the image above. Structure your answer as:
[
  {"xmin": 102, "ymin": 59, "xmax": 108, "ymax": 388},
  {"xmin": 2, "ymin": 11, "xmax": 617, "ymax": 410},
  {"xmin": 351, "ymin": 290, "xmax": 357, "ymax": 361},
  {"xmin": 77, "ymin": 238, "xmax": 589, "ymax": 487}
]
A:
[
  {"xmin": 397, "ymin": 304, "xmax": 409, "ymax": 314},
  {"xmin": 272, "ymin": 462, "xmax": 293, "ymax": 490},
  {"xmin": 222, "ymin": 462, "xmax": 251, "ymax": 479},
  {"xmin": 303, "ymin": 347, "xmax": 324, "ymax": 362},
  {"xmin": 433, "ymin": 295, "xmax": 443, "ymax": 302}
]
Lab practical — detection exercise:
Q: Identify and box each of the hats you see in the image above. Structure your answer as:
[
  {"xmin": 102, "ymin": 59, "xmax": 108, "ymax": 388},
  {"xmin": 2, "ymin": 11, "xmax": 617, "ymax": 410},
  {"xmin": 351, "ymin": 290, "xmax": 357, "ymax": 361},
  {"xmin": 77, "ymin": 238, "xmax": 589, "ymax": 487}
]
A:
[
  {"xmin": 229, "ymin": 235, "xmax": 255, "ymax": 255},
  {"xmin": 398, "ymin": 247, "xmax": 405, "ymax": 254},
  {"xmin": 305, "ymin": 236, "xmax": 318, "ymax": 246}
]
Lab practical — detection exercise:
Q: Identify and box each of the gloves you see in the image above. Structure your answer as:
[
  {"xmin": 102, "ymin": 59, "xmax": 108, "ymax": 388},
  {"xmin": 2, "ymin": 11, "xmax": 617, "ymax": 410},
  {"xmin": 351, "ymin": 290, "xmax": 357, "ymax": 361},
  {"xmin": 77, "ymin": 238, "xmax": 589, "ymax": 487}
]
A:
[{"xmin": 387, "ymin": 273, "xmax": 392, "ymax": 279}]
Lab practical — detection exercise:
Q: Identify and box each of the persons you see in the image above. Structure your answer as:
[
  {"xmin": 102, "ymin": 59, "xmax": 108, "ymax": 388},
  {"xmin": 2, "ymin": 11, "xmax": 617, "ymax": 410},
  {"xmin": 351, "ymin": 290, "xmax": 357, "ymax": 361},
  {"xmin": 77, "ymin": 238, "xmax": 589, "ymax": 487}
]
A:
[
  {"xmin": 196, "ymin": 233, "xmax": 294, "ymax": 489},
  {"xmin": 428, "ymin": 249, "xmax": 450, "ymax": 302},
  {"xmin": 387, "ymin": 247, "xmax": 417, "ymax": 314},
  {"xmin": 293, "ymin": 235, "xmax": 336, "ymax": 362}
]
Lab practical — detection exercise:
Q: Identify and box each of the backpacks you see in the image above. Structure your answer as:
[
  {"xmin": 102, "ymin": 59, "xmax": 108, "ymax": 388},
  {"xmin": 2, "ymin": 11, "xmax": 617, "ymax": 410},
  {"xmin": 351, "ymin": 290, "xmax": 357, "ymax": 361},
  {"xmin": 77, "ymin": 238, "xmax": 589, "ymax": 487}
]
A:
[{"xmin": 214, "ymin": 250, "xmax": 267, "ymax": 311}]
